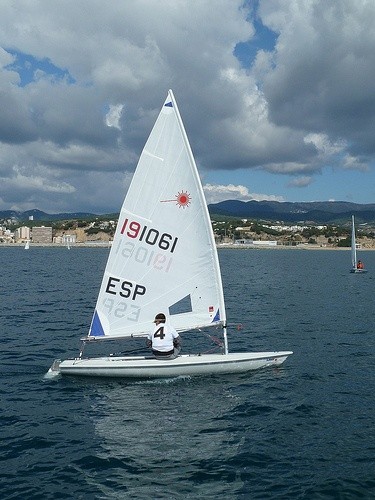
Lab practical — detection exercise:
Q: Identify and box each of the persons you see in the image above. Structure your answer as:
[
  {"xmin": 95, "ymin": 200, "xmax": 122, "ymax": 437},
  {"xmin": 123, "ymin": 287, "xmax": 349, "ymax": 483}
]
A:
[
  {"xmin": 357, "ymin": 260, "xmax": 364, "ymax": 268},
  {"xmin": 147, "ymin": 313, "xmax": 182, "ymax": 360}
]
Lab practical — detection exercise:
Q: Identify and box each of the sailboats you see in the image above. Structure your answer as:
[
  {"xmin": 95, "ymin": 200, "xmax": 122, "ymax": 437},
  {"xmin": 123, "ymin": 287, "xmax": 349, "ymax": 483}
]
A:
[
  {"xmin": 350, "ymin": 215, "xmax": 368, "ymax": 274},
  {"xmin": 46, "ymin": 88, "xmax": 293, "ymax": 382}
]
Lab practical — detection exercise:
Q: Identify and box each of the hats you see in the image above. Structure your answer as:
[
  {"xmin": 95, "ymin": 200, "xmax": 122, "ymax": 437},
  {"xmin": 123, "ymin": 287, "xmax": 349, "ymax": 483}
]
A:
[{"xmin": 153, "ymin": 313, "xmax": 166, "ymax": 323}]
